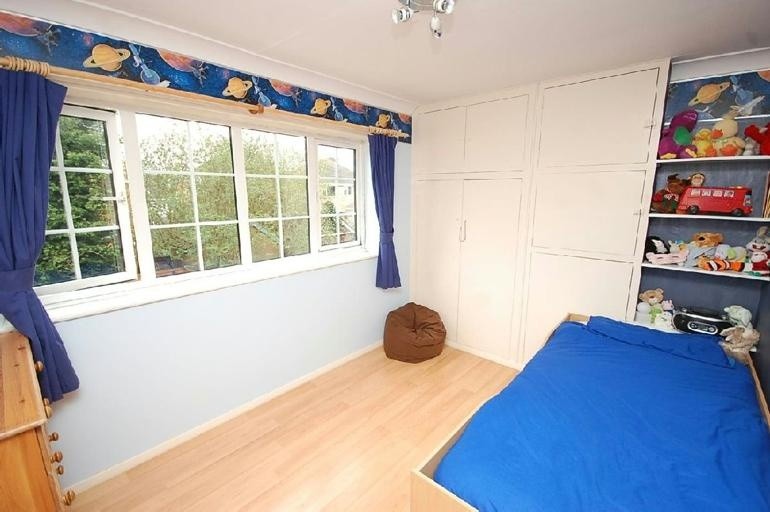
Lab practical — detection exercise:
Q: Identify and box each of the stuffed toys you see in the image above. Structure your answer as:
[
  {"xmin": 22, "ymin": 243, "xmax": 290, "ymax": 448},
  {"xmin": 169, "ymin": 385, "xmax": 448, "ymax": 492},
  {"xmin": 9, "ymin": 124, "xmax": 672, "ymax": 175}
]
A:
[{"xmin": 636, "ymin": 110, "xmax": 770, "ymax": 353}]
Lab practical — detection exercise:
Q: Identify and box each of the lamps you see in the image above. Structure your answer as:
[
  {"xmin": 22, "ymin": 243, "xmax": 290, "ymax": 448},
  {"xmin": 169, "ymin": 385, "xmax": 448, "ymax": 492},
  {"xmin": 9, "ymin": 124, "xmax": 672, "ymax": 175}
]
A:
[{"xmin": 390, "ymin": 0, "xmax": 457, "ymax": 37}]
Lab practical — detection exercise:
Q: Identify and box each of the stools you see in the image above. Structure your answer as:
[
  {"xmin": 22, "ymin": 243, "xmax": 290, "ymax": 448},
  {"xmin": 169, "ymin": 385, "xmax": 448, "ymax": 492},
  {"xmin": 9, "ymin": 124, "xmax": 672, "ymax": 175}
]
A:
[{"xmin": 384, "ymin": 298, "xmax": 445, "ymax": 365}]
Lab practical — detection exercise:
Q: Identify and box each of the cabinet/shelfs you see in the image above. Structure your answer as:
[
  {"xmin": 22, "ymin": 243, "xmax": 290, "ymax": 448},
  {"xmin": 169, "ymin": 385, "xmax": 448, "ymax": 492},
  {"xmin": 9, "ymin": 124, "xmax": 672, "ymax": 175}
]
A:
[
  {"xmin": 1, "ymin": 321, "xmax": 77, "ymax": 511},
  {"xmin": 630, "ymin": 44, "xmax": 769, "ymax": 371},
  {"xmin": 408, "ymin": 169, "xmax": 532, "ymax": 367},
  {"xmin": 522, "ymin": 57, "xmax": 670, "ymax": 370},
  {"xmin": 413, "ymin": 83, "xmax": 538, "ymax": 168}
]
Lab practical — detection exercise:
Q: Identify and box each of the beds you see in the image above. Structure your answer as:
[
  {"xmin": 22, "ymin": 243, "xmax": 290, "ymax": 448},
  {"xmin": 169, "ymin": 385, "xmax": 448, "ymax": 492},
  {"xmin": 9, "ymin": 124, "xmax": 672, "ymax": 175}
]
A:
[{"xmin": 412, "ymin": 310, "xmax": 770, "ymax": 512}]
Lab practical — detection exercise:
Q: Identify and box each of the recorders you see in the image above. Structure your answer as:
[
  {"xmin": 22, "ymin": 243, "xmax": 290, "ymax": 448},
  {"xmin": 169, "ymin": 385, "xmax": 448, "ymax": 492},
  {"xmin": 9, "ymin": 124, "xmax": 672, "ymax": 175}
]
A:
[{"xmin": 672, "ymin": 305, "xmax": 738, "ymax": 337}]
